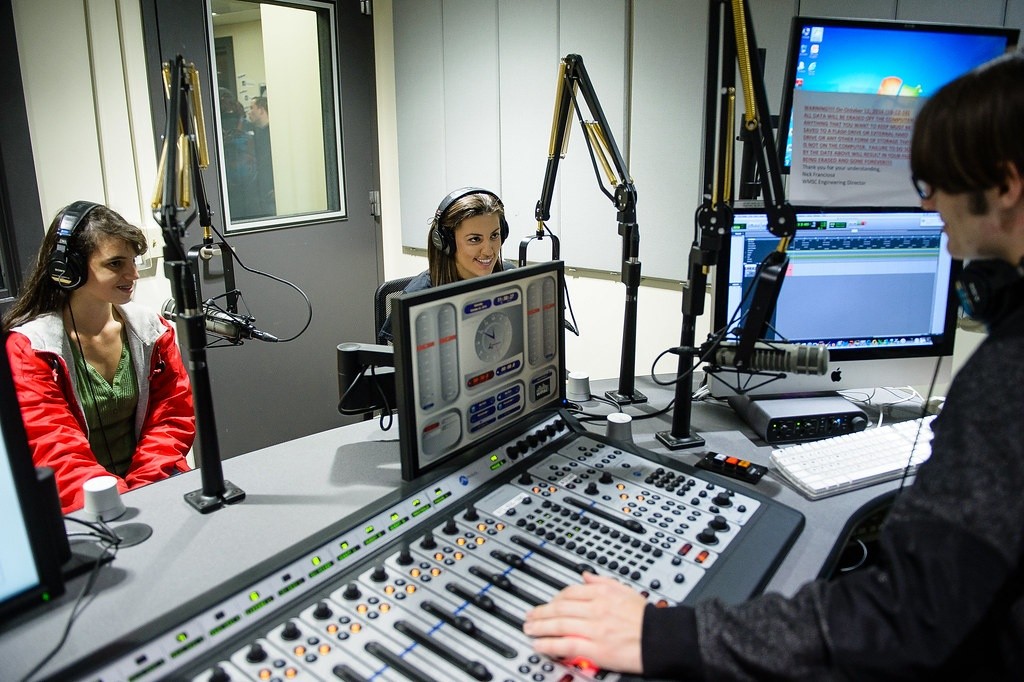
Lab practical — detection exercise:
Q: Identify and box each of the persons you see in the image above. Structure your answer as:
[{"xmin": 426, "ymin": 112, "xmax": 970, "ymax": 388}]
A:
[
  {"xmin": 376, "ymin": 186, "xmax": 516, "ymax": 342},
  {"xmin": 218, "ymin": 87, "xmax": 276, "ymax": 221},
  {"xmin": 1, "ymin": 200, "xmax": 196, "ymax": 514},
  {"xmin": 524, "ymin": 51, "xmax": 1024, "ymax": 682}
]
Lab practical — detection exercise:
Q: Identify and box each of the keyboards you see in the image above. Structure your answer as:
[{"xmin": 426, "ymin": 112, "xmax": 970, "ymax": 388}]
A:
[{"xmin": 769, "ymin": 414, "xmax": 938, "ymax": 501}]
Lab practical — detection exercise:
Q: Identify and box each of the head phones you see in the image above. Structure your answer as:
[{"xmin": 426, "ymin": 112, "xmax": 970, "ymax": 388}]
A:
[
  {"xmin": 47, "ymin": 203, "xmax": 102, "ymax": 292},
  {"xmin": 433, "ymin": 187, "xmax": 509, "ymax": 255},
  {"xmin": 956, "ymin": 254, "xmax": 1024, "ymax": 329}
]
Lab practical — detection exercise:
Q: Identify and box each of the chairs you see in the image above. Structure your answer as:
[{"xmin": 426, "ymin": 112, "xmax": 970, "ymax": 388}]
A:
[{"xmin": 375, "ymin": 262, "xmax": 517, "ymax": 345}]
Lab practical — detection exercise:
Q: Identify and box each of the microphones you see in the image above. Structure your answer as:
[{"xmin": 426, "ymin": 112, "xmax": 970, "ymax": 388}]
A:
[
  {"xmin": 671, "ymin": 342, "xmax": 829, "ymax": 376},
  {"xmin": 162, "ymin": 298, "xmax": 278, "ymax": 345}
]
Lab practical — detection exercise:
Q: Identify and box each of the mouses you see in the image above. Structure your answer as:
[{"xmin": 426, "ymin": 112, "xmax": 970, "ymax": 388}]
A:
[{"xmin": 920, "ymin": 396, "xmax": 945, "ymax": 415}]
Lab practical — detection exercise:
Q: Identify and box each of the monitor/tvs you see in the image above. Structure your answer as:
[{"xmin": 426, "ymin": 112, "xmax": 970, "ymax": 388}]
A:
[
  {"xmin": 776, "ymin": 16, "xmax": 1019, "ymax": 204},
  {"xmin": 0, "ymin": 329, "xmax": 66, "ymax": 627},
  {"xmin": 709, "ymin": 206, "xmax": 957, "ymax": 398},
  {"xmin": 392, "ymin": 260, "xmax": 566, "ymax": 481}
]
